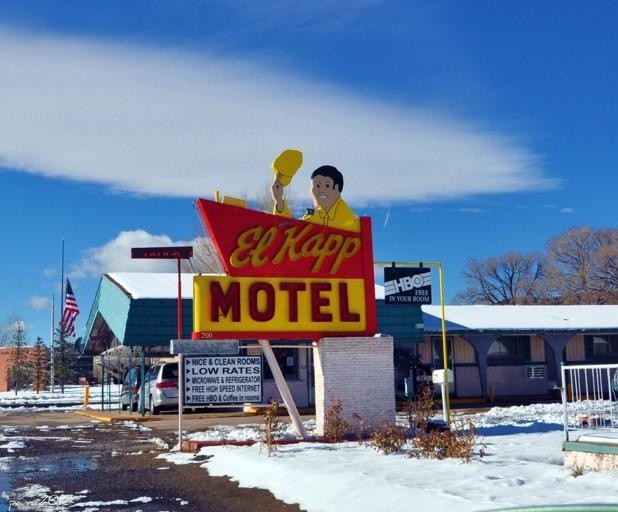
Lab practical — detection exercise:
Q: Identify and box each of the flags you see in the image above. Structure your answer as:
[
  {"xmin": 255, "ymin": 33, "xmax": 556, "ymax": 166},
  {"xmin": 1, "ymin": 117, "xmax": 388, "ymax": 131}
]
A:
[{"xmin": 60, "ymin": 276, "xmax": 79, "ymax": 339}]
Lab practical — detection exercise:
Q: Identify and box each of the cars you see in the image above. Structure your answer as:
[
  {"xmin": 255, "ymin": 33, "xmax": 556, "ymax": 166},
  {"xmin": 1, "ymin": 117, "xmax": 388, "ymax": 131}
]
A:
[
  {"xmin": 138, "ymin": 361, "xmax": 179, "ymax": 415},
  {"xmin": 120, "ymin": 365, "xmax": 154, "ymax": 412}
]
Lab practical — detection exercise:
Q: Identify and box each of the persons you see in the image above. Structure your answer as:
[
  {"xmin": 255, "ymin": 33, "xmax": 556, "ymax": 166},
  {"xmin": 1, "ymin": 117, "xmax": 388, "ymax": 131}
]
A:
[{"xmin": 271, "ymin": 164, "xmax": 361, "ymax": 231}]
